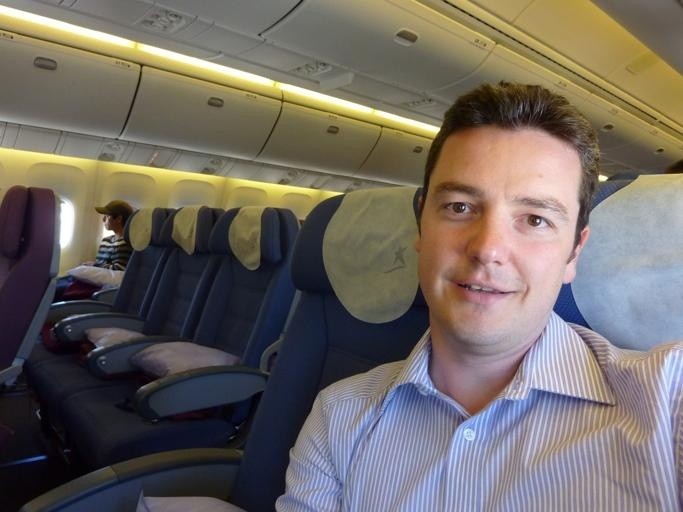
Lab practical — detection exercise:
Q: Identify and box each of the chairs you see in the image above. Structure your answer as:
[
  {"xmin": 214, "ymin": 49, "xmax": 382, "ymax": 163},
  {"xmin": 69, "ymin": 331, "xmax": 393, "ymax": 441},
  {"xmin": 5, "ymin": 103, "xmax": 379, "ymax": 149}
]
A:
[
  {"xmin": 18, "ymin": 186, "xmax": 427, "ymax": 512},
  {"xmin": 0, "ymin": 184, "xmax": 61, "ymax": 439},
  {"xmin": 552, "ymin": 174, "xmax": 683, "ymax": 352}
]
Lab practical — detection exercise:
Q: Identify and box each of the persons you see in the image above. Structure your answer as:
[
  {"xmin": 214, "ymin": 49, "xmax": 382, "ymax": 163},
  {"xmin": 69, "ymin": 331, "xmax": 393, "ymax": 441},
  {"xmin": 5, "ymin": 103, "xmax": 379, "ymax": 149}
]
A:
[
  {"xmin": 274, "ymin": 78, "xmax": 683, "ymax": 512},
  {"xmin": 52, "ymin": 199, "xmax": 133, "ymax": 304}
]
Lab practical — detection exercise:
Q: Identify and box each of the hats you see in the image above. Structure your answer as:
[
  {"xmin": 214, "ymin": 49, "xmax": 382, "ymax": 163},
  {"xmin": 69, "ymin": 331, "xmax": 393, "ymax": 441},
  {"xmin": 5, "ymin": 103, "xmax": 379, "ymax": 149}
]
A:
[{"xmin": 94, "ymin": 200, "xmax": 133, "ymax": 216}]
